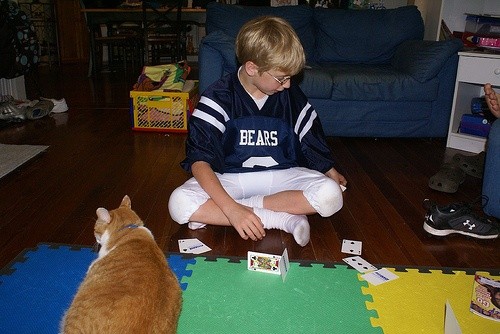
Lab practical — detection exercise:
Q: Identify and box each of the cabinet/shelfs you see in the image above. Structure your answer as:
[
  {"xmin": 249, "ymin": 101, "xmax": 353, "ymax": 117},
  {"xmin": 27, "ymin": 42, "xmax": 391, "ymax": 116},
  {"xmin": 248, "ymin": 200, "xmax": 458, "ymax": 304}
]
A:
[{"xmin": 18, "ymin": 0, "xmax": 61, "ymax": 72}]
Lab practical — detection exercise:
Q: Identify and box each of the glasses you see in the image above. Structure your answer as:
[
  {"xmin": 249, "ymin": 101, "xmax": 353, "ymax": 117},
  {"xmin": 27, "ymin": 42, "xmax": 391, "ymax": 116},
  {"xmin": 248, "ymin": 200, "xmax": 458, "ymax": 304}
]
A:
[{"xmin": 265, "ymin": 71, "xmax": 291, "ymax": 86}]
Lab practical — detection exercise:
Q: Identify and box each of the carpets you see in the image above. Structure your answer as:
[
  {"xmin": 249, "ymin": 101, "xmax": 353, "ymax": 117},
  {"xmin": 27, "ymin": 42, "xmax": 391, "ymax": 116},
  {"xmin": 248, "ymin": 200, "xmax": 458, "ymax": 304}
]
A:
[
  {"xmin": 0, "ymin": 242, "xmax": 500, "ymax": 334},
  {"xmin": 0, "ymin": 143, "xmax": 51, "ymax": 180}
]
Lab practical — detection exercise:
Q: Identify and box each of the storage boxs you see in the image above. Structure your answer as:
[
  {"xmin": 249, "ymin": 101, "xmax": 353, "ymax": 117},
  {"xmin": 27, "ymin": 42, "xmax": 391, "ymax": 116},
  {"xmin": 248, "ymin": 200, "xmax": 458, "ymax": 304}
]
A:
[
  {"xmin": 465, "ymin": 16, "xmax": 500, "ymax": 36},
  {"xmin": 457, "ymin": 113, "xmax": 493, "ymax": 139},
  {"xmin": 127, "ymin": 79, "xmax": 199, "ymax": 134}
]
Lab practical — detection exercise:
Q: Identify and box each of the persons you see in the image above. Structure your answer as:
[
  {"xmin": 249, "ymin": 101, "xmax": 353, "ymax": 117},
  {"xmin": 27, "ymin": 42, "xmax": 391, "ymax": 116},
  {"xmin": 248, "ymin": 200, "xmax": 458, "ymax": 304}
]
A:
[
  {"xmin": 167, "ymin": 15, "xmax": 348, "ymax": 247},
  {"xmin": 482, "ymin": 83, "xmax": 500, "ymax": 225}
]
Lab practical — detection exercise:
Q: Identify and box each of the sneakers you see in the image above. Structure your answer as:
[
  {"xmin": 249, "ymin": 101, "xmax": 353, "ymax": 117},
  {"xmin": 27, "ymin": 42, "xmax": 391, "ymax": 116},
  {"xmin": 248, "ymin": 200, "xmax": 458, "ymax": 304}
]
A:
[
  {"xmin": 422, "ymin": 195, "xmax": 500, "ymax": 239},
  {"xmin": 40, "ymin": 97, "xmax": 69, "ymax": 113},
  {"xmin": 0, "ymin": 100, "xmax": 25, "ymax": 120},
  {"xmin": 25, "ymin": 100, "xmax": 54, "ymax": 119}
]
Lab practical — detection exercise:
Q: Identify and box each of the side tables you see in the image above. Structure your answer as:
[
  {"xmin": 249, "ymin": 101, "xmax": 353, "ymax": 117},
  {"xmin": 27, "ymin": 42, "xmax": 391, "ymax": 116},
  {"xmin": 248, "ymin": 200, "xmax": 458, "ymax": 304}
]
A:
[{"xmin": 445, "ymin": 51, "xmax": 500, "ymax": 154}]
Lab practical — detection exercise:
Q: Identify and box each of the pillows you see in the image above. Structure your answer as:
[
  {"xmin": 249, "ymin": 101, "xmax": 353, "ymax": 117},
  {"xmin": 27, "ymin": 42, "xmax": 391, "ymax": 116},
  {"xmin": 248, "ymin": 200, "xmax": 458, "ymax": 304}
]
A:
[
  {"xmin": 391, "ymin": 37, "xmax": 463, "ymax": 85},
  {"xmin": 200, "ymin": 27, "xmax": 238, "ymax": 72}
]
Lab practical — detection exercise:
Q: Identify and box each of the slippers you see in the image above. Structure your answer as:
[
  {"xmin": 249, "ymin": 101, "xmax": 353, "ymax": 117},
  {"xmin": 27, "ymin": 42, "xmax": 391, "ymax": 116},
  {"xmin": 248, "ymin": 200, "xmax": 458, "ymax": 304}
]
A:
[
  {"xmin": 429, "ymin": 167, "xmax": 468, "ymax": 193},
  {"xmin": 453, "ymin": 152, "xmax": 484, "ymax": 179}
]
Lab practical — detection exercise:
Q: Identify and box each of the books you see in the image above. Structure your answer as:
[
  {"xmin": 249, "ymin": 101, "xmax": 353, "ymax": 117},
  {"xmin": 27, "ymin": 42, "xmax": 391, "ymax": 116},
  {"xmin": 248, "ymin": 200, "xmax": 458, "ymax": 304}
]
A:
[{"xmin": 471, "ymin": 274, "xmax": 500, "ymax": 322}]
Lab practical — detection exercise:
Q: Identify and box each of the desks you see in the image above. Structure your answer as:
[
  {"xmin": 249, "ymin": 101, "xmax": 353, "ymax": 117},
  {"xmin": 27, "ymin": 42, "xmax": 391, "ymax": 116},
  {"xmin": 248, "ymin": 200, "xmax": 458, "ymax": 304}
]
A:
[{"xmin": 80, "ymin": 8, "xmax": 207, "ymax": 81}]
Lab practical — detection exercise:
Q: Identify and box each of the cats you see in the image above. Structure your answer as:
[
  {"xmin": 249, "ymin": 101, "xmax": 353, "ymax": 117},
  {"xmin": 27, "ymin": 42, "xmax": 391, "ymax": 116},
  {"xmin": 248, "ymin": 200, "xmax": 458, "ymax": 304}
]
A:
[{"xmin": 58, "ymin": 194, "xmax": 183, "ymax": 334}]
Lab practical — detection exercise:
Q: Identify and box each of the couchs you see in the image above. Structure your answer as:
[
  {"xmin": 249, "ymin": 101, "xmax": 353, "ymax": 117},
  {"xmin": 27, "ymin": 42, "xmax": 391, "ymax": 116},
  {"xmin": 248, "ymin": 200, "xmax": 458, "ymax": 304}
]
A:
[{"xmin": 198, "ymin": 3, "xmax": 464, "ymax": 145}]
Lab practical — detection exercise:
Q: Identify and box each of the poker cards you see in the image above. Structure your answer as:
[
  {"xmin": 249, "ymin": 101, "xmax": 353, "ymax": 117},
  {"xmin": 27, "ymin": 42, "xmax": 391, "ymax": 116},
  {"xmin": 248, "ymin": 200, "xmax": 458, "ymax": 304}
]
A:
[
  {"xmin": 248, "ymin": 251, "xmax": 282, "ymax": 275},
  {"xmin": 361, "ymin": 268, "xmax": 399, "ymax": 286},
  {"xmin": 340, "ymin": 184, "xmax": 346, "ymax": 193},
  {"xmin": 279, "ymin": 248, "xmax": 290, "ymax": 284},
  {"xmin": 341, "ymin": 239, "xmax": 362, "ymax": 255},
  {"xmin": 342, "ymin": 256, "xmax": 378, "ymax": 273},
  {"xmin": 178, "ymin": 238, "xmax": 212, "ymax": 255}
]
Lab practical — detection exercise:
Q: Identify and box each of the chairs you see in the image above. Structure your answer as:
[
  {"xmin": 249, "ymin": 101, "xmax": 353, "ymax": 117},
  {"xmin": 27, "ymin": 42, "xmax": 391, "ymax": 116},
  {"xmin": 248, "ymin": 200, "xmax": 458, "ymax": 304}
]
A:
[
  {"xmin": 105, "ymin": 0, "xmax": 144, "ymax": 74},
  {"xmin": 79, "ymin": 0, "xmax": 138, "ymax": 81},
  {"xmin": 141, "ymin": 0, "xmax": 181, "ymax": 65},
  {"xmin": 151, "ymin": 0, "xmax": 192, "ymax": 66}
]
